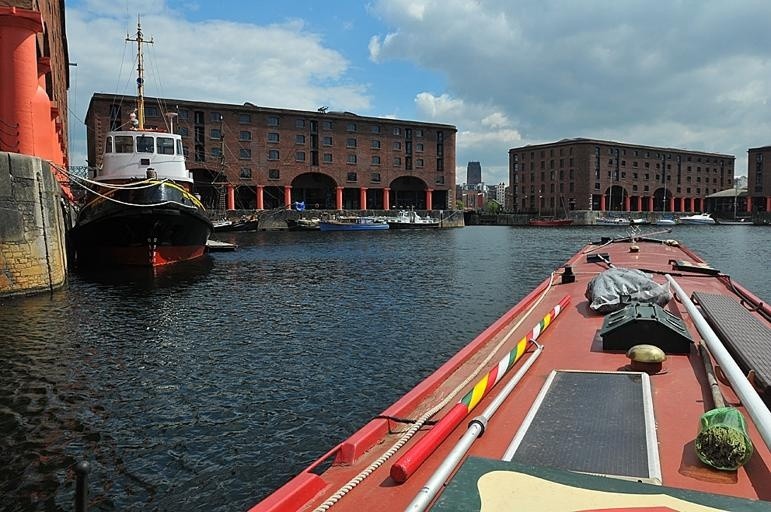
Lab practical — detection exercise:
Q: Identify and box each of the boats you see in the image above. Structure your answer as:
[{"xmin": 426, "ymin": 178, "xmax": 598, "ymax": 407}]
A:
[
  {"xmin": 284, "ymin": 210, "xmax": 440, "ymax": 232},
  {"xmin": 528, "ymin": 215, "xmax": 573, "ymax": 226},
  {"xmin": 596, "ymin": 213, "xmax": 753, "ymax": 225}
]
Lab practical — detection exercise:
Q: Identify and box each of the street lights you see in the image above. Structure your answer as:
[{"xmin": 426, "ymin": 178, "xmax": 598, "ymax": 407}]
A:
[
  {"xmin": 733, "ymin": 183, "xmax": 738, "ymax": 218},
  {"xmin": 538, "ymin": 191, "xmax": 543, "ymax": 216}
]
